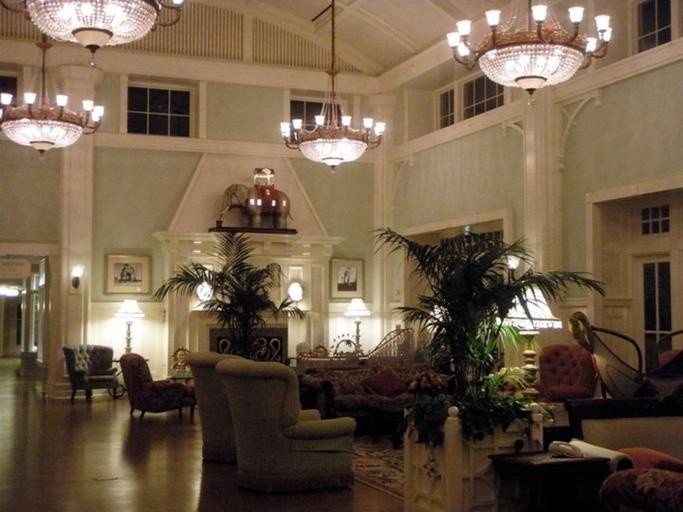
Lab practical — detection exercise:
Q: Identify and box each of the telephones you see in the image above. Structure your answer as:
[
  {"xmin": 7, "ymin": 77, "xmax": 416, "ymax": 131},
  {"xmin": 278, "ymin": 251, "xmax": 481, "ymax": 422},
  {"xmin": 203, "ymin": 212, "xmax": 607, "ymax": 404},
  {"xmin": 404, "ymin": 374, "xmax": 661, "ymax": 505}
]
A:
[{"xmin": 549, "ymin": 440, "xmax": 584, "ymax": 458}]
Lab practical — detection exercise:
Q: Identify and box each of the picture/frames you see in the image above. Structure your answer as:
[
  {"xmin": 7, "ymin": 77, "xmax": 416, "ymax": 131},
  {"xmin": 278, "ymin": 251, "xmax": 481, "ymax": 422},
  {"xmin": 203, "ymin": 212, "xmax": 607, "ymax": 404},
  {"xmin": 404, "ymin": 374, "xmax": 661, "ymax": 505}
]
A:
[
  {"xmin": 329, "ymin": 258, "xmax": 365, "ymax": 301},
  {"xmin": 103, "ymin": 253, "xmax": 153, "ymax": 295}
]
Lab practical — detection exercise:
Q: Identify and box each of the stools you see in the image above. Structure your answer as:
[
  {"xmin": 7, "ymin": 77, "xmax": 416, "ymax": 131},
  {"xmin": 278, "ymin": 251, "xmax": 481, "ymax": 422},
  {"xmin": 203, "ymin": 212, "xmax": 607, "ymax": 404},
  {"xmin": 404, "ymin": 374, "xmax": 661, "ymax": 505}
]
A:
[{"xmin": 619, "ymin": 446, "xmax": 683, "ymax": 473}]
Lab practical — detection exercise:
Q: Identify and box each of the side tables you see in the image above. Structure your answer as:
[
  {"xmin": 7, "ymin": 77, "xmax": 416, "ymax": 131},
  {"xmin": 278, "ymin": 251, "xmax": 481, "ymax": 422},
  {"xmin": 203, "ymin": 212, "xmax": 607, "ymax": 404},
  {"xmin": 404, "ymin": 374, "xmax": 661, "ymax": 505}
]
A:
[{"xmin": 487, "ymin": 450, "xmax": 610, "ymax": 512}]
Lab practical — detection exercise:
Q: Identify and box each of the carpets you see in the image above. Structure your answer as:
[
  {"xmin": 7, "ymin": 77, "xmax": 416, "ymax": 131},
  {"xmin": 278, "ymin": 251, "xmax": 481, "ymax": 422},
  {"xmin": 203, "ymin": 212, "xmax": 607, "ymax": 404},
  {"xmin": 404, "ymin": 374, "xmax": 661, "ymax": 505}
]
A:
[{"xmin": 350, "ymin": 437, "xmax": 405, "ymax": 501}]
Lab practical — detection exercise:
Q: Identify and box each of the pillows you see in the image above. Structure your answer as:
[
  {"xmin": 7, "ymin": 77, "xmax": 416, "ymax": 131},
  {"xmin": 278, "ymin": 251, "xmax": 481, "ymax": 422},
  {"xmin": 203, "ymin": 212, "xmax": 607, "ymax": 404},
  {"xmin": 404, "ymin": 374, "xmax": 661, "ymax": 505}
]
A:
[{"xmin": 364, "ymin": 368, "xmax": 407, "ymax": 398}]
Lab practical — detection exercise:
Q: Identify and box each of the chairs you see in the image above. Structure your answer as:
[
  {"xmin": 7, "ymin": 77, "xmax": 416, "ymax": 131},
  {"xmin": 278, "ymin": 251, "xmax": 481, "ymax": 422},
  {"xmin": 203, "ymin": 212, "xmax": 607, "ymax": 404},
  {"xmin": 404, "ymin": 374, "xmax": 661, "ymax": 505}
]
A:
[
  {"xmin": 120, "ymin": 354, "xmax": 198, "ymax": 419},
  {"xmin": 63, "ymin": 344, "xmax": 119, "ymax": 403},
  {"xmin": 505, "ymin": 344, "xmax": 598, "ymax": 404},
  {"xmin": 217, "ymin": 358, "xmax": 356, "ymax": 494},
  {"xmin": 185, "ymin": 351, "xmax": 241, "ymax": 464}
]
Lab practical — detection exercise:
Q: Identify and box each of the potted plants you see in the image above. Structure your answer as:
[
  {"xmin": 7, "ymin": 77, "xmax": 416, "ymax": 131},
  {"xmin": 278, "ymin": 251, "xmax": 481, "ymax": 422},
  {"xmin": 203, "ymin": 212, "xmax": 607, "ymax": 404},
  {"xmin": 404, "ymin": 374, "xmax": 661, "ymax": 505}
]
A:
[{"xmin": 369, "ymin": 227, "xmax": 605, "ymax": 512}]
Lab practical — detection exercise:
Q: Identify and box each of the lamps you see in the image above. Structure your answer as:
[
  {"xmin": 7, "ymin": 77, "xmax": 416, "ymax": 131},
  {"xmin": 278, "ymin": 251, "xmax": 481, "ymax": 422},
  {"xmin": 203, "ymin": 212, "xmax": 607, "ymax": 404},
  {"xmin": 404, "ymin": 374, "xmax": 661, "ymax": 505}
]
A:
[
  {"xmin": 114, "ymin": 299, "xmax": 145, "ymax": 354},
  {"xmin": 280, "ymin": 0, "xmax": 387, "ymax": 174},
  {"xmin": 70, "ymin": 264, "xmax": 85, "ymax": 289},
  {"xmin": 344, "ymin": 298, "xmax": 373, "ymax": 345},
  {"xmin": 447, "ymin": 0, "xmax": 612, "ymax": 107},
  {"xmin": 0, "ymin": 34, "xmax": 104, "ymax": 160},
  {"xmin": 506, "ymin": 253, "xmax": 521, "ymax": 282},
  {"xmin": 502, "ymin": 282, "xmax": 564, "ymax": 395},
  {"xmin": 0, "ymin": 0, "xmax": 183, "ymax": 67}
]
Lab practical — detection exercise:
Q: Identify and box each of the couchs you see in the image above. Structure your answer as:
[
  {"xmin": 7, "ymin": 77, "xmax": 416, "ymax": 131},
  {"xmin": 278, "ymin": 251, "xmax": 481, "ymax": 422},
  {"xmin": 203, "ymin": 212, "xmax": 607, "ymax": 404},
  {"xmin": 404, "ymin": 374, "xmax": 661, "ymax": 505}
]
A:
[{"xmin": 301, "ymin": 363, "xmax": 429, "ymax": 437}]
